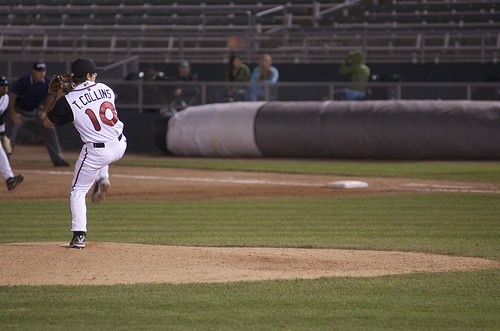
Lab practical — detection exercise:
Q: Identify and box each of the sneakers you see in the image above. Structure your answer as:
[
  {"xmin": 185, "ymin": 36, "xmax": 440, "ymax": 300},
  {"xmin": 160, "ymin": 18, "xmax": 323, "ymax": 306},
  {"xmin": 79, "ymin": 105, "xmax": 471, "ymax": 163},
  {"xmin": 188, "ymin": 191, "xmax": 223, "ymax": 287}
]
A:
[
  {"xmin": 70, "ymin": 235, "xmax": 86, "ymax": 248},
  {"xmin": 92, "ymin": 179, "xmax": 110, "ymax": 202}
]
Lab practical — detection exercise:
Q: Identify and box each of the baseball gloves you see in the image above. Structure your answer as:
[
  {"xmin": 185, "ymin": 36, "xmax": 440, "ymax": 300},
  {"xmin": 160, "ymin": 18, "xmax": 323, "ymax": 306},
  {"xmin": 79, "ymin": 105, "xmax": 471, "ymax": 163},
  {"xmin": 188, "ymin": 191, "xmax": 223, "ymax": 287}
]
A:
[{"xmin": 48, "ymin": 72, "xmax": 74, "ymax": 95}]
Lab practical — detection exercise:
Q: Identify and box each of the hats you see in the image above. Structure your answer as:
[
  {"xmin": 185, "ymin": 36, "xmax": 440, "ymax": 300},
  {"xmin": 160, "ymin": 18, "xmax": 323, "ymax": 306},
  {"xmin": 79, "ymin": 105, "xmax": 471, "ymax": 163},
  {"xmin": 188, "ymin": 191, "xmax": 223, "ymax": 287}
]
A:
[
  {"xmin": 32, "ymin": 62, "xmax": 47, "ymax": 71},
  {"xmin": 0, "ymin": 77, "xmax": 8, "ymax": 85},
  {"xmin": 71, "ymin": 58, "xmax": 104, "ymax": 77}
]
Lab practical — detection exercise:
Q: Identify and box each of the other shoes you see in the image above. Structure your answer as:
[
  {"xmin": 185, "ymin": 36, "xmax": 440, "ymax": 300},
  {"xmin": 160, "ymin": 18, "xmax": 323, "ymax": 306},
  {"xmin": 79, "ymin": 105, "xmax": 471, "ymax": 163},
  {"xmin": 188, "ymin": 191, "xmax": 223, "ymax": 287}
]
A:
[
  {"xmin": 6, "ymin": 175, "xmax": 24, "ymax": 191},
  {"xmin": 54, "ymin": 159, "xmax": 69, "ymax": 167}
]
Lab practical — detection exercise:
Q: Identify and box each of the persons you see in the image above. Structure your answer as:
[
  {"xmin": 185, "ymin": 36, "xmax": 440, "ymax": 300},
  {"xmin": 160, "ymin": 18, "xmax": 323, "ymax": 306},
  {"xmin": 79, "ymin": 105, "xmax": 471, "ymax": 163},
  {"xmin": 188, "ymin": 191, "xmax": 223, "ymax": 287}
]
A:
[
  {"xmin": 225, "ymin": 56, "xmax": 251, "ymax": 101},
  {"xmin": 2, "ymin": 59, "xmax": 71, "ymax": 167},
  {"xmin": 243, "ymin": 55, "xmax": 279, "ymax": 100},
  {"xmin": 0, "ymin": 77, "xmax": 26, "ymax": 192},
  {"xmin": 324, "ymin": 50, "xmax": 370, "ymax": 101},
  {"xmin": 43, "ymin": 58, "xmax": 128, "ymax": 248},
  {"xmin": 166, "ymin": 57, "xmax": 201, "ymax": 108}
]
